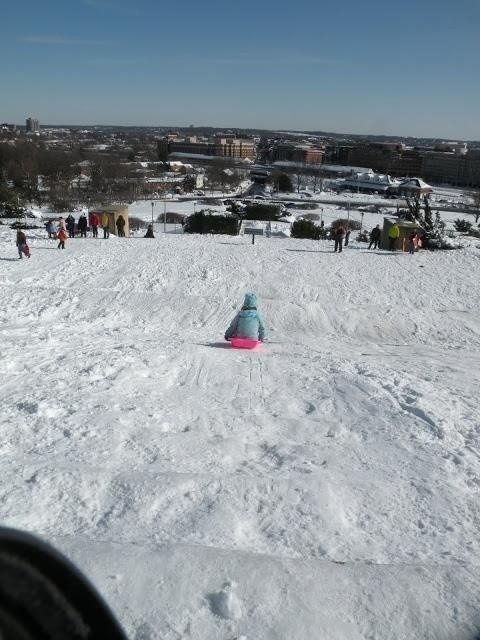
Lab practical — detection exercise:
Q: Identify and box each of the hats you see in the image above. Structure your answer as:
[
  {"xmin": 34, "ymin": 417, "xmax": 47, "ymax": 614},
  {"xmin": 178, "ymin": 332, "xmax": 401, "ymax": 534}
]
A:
[{"xmin": 243, "ymin": 293, "xmax": 258, "ymax": 307}]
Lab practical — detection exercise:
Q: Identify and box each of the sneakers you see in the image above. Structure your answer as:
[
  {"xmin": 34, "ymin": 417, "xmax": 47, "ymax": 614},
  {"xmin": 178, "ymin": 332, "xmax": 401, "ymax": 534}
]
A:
[
  {"xmin": 49, "ymin": 234, "xmax": 110, "ymax": 238},
  {"xmin": 58, "ymin": 245, "xmax": 66, "ymax": 249}
]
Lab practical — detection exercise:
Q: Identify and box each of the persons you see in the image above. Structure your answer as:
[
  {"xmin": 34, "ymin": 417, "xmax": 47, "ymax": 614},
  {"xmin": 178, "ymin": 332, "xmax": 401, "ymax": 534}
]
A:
[
  {"xmin": 16, "ymin": 227, "xmax": 31, "ymax": 259},
  {"xmin": 335, "ymin": 224, "xmax": 351, "ymax": 252},
  {"xmin": 368, "ymin": 223, "xmax": 422, "ymax": 254},
  {"xmin": 224, "ymin": 292, "xmax": 267, "ymax": 341},
  {"xmin": 46, "ymin": 211, "xmax": 125, "ymax": 250}
]
